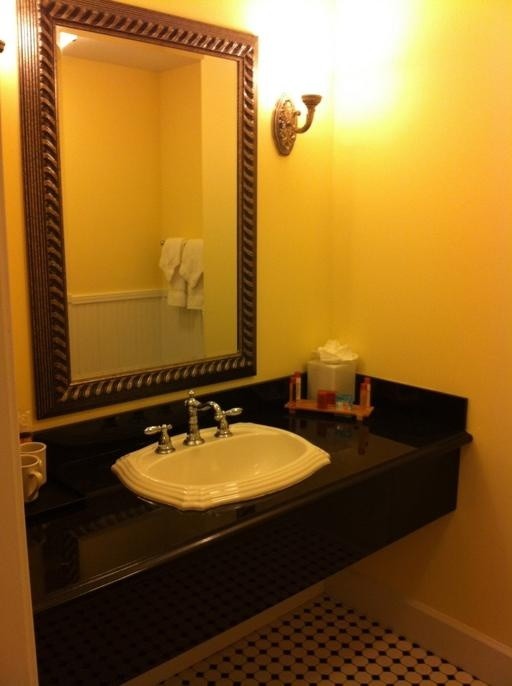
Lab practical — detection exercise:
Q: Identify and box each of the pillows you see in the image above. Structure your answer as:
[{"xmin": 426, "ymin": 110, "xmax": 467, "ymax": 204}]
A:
[{"xmin": 159, "ymin": 238, "xmax": 203, "ymax": 310}]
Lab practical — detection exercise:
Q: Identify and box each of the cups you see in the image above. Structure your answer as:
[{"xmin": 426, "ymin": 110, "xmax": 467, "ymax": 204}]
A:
[
  {"xmin": 20, "ymin": 454, "xmax": 44, "ymax": 504},
  {"xmin": 19, "ymin": 440, "xmax": 49, "ymax": 487}
]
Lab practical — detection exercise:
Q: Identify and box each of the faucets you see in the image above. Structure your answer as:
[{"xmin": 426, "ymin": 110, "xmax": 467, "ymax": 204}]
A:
[{"xmin": 184, "ymin": 390, "xmax": 224, "ymax": 445}]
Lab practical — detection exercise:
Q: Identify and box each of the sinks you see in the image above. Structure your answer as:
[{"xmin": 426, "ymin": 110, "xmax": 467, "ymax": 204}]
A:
[{"xmin": 112, "ymin": 422, "xmax": 331, "ymax": 512}]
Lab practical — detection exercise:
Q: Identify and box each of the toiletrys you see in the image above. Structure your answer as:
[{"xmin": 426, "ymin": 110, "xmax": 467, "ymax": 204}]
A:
[
  {"xmin": 294, "ymin": 372, "xmax": 302, "ymax": 401},
  {"xmin": 364, "ymin": 378, "xmax": 373, "ymax": 406},
  {"xmin": 316, "ymin": 389, "xmax": 336, "ymax": 409},
  {"xmin": 356, "ymin": 424, "xmax": 368, "ymax": 454},
  {"xmin": 289, "ymin": 378, "xmax": 297, "ymax": 404},
  {"xmin": 360, "ymin": 381, "xmax": 367, "ymax": 407}
]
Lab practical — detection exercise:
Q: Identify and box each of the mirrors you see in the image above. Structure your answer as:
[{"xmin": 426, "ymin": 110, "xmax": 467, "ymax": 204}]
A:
[{"xmin": 17, "ymin": 0, "xmax": 258, "ymax": 420}]
